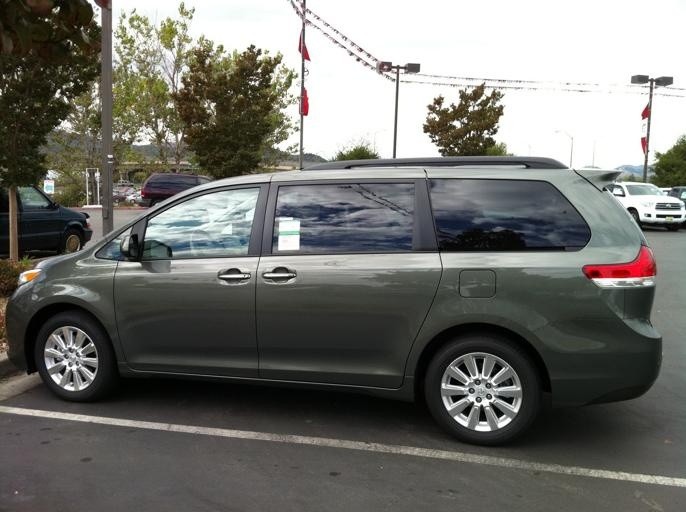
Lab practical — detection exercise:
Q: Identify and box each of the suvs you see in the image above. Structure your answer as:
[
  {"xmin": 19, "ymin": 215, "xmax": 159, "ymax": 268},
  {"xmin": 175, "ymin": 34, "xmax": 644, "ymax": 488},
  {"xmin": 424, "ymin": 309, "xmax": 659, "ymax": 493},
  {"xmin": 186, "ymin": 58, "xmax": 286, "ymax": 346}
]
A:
[
  {"xmin": 612, "ymin": 181, "xmax": 686, "ymax": 232},
  {"xmin": 0, "ymin": 180, "xmax": 92, "ymax": 256}
]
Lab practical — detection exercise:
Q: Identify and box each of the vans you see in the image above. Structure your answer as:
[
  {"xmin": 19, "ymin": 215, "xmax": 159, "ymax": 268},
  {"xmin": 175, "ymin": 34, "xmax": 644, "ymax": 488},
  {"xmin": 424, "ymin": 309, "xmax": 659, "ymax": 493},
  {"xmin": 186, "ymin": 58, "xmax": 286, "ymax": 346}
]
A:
[
  {"xmin": 142, "ymin": 173, "xmax": 215, "ymax": 208},
  {"xmin": 4, "ymin": 153, "xmax": 666, "ymax": 445}
]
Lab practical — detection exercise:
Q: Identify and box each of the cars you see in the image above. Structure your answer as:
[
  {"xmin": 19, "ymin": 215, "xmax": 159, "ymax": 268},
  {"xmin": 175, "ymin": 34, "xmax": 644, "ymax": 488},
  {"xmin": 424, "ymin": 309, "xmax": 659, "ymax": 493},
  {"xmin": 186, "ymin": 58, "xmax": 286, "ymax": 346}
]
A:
[{"xmin": 111, "ymin": 177, "xmax": 151, "ymax": 208}]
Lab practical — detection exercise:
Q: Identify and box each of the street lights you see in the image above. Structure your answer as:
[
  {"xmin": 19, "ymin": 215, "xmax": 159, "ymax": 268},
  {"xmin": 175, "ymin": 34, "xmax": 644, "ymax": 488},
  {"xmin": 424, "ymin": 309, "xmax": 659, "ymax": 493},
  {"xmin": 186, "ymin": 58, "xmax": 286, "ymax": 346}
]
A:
[
  {"xmin": 375, "ymin": 61, "xmax": 421, "ymax": 158},
  {"xmin": 630, "ymin": 75, "xmax": 674, "ymax": 183},
  {"xmin": 555, "ymin": 129, "xmax": 574, "ymax": 168}
]
